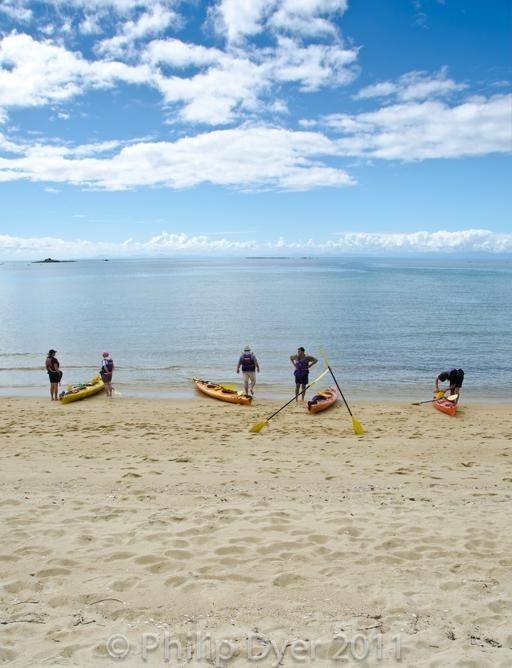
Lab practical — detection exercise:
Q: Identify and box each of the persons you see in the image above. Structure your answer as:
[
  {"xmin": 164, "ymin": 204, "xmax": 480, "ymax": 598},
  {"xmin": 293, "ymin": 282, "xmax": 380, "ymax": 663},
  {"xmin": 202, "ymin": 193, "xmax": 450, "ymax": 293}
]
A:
[
  {"xmin": 235, "ymin": 345, "xmax": 261, "ymax": 397},
  {"xmin": 46, "ymin": 350, "xmax": 63, "ymax": 402},
  {"xmin": 290, "ymin": 347, "xmax": 318, "ymax": 401},
  {"xmin": 100, "ymin": 352, "xmax": 114, "ymax": 397},
  {"xmin": 434, "ymin": 368, "xmax": 464, "ymax": 406}
]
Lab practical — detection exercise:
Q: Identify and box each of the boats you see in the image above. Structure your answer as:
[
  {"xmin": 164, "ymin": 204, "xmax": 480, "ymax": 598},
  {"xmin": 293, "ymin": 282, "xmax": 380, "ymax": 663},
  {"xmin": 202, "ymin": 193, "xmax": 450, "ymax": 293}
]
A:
[
  {"xmin": 196, "ymin": 378, "xmax": 251, "ymax": 404},
  {"xmin": 62, "ymin": 376, "xmax": 105, "ymax": 404},
  {"xmin": 433, "ymin": 393, "xmax": 456, "ymax": 416},
  {"xmin": 309, "ymin": 386, "xmax": 338, "ymax": 413}
]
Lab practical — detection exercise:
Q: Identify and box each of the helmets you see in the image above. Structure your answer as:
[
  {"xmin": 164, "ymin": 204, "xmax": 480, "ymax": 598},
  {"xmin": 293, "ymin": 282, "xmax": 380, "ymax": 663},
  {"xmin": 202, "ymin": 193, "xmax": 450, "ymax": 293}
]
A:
[{"xmin": 103, "ymin": 352, "xmax": 109, "ymax": 357}]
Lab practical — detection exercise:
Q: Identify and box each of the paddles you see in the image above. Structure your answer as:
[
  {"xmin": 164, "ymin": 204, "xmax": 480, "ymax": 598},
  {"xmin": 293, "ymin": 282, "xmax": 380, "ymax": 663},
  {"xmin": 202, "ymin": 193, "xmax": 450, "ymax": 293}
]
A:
[
  {"xmin": 319, "ymin": 347, "xmax": 363, "ymax": 436},
  {"xmin": 193, "ymin": 378, "xmax": 236, "ymax": 391},
  {"xmin": 66, "ymin": 383, "xmax": 80, "ymax": 390},
  {"xmin": 248, "ymin": 367, "xmax": 329, "ymax": 432},
  {"xmin": 410, "ymin": 393, "xmax": 459, "ymax": 406}
]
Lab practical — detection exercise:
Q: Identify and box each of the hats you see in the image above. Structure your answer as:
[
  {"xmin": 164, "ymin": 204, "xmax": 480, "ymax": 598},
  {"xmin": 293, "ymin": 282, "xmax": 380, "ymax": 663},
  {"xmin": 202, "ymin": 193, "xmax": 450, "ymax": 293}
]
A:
[
  {"xmin": 49, "ymin": 350, "xmax": 57, "ymax": 354},
  {"xmin": 243, "ymin": 346, "xmax": 250, "ymax": 351}
]
